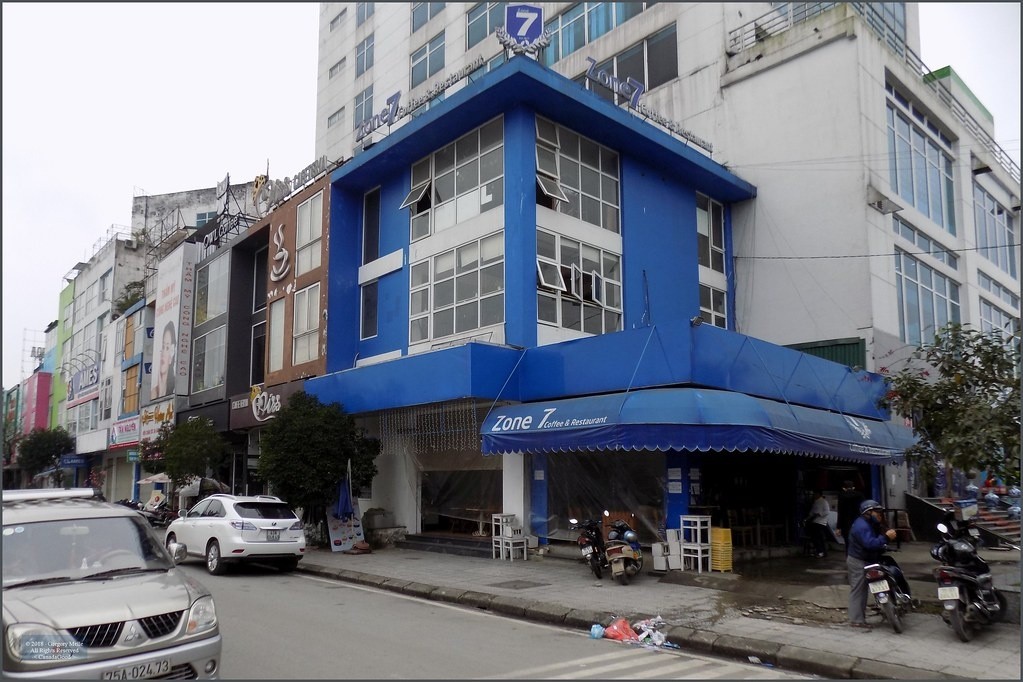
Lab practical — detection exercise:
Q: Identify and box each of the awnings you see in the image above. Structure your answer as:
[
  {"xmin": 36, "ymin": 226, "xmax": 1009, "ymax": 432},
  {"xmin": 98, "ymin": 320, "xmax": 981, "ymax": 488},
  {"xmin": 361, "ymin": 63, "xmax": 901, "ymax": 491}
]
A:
[
  {"xmin": 34, "ymin": 454, "xmax": 89, "ymax": 478},
  {"xmin": 477, "ymin": 388, "xmax": 938, "ymax": 470}
]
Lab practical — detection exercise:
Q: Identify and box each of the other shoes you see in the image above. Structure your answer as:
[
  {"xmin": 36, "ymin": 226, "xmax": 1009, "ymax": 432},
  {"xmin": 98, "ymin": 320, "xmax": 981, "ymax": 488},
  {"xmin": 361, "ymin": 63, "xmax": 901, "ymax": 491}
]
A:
[{"xmin": 850, "ymin": 623, "xmax": 872, "ymax": 628}]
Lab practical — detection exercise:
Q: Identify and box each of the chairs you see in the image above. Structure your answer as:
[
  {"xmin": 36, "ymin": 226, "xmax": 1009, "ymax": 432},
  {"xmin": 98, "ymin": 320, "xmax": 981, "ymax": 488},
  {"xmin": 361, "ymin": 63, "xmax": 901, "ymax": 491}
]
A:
[{"xmin": 728, "ymin": 508, "xmax": 776, "ymax": 548}]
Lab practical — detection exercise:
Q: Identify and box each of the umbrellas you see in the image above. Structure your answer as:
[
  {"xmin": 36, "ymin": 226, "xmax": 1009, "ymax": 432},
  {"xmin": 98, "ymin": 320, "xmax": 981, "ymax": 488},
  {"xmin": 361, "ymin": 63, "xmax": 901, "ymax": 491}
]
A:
[
  {"xmin": 137, "ymin": 470, "xmax": 196, "ymax": 511},
  {"xmin": 330, "ymin": 466, "xmax": 352, "ymax": 523},
  {"xmin": 175, "ymin": 476, "xmax": 230, "ymax": 499}
]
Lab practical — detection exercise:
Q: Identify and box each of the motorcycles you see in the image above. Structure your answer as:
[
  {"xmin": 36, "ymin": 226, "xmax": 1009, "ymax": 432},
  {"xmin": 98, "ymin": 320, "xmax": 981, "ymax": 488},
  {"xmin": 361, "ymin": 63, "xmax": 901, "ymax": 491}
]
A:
[
  {"xmin": 568, "ymin": 518, "xmax": 607, "ymax": 579},
  {"xmin": 863, "ymin": 544, "xmax": 921, "ymax": 634}
]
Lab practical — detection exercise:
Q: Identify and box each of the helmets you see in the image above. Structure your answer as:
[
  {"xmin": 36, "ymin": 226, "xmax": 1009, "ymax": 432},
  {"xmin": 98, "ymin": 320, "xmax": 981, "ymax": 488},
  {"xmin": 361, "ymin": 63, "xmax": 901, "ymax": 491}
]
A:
[
  {"xmin": 930, "ymin": 541, "xmax": 949, "ymax": 562},
  {"xmin": 625, "ymin": 530, "xmax": 638, "ymax": 542},
  {"xmin": 860, "ymin": 500, "xmax": 884, "ymax": 515},
  {"xmin": 608, "ymin": 531, "xmax": 619, "ymax": 540}
]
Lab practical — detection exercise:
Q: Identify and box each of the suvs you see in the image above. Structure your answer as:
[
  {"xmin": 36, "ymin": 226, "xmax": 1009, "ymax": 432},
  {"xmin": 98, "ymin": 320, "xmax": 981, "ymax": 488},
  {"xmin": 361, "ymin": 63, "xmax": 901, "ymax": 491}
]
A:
[
  {"xmin": 163, "ymin": 491, "xmax": 306, "ymax": 575},
  {"xmin": 0, "ymin": 485, "xmax": 222, "ymax": 682}
]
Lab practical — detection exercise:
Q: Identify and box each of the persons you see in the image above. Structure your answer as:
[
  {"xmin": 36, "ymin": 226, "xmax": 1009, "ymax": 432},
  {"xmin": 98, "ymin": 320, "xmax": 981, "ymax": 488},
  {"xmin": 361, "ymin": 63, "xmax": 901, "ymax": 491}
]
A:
[
  {"xmin": 834, "ymin": 481, "xmax": 864, "ymax": 567},
  {"xmin": 803, "ymin": 487, "xmax": 830, "ymax": 559},
  {"xmin": 153, "ymin": 322, "xmax": 177, "ymax": 398},
  {"xmin": 845, "ymin": 501, "xmax": 896, "ymax": 626}
]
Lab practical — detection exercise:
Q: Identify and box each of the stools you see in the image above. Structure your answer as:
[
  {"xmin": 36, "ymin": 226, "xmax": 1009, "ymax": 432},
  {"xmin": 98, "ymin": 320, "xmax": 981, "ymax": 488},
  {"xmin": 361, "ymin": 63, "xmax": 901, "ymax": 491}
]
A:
[
  {"xmin": 680, "ymin": 515, "xmax": 712, "ymax": 573},
  {"xmin": 492, "ymin": 513, "xmax": 527, "ymax": 562}
]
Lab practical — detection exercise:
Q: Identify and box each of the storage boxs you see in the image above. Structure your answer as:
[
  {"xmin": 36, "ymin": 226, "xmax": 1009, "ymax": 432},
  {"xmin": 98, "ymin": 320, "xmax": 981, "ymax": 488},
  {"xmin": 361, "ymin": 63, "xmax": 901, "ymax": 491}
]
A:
[
  {"xmin": 711, "ymin": 526, "xmax": 733, "ymax": 573},
  {"xmin": 366, "ymin": 511, "xmax": 394, "ymax": 530}
]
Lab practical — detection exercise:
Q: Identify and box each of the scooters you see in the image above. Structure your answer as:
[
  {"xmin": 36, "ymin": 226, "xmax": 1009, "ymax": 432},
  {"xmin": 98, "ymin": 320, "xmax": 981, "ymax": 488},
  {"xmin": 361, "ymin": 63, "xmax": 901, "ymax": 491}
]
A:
[
  {"xmin": 929, "ymin": 522, "xmax": 1007, "ymax": 643},
  {"xmin": 603, "ymin": 509, "xmax": 643, "ymax": 586},
  {"xmin": 115, "ymin": 498, "xmax": 179, "ymax": 529}
]
